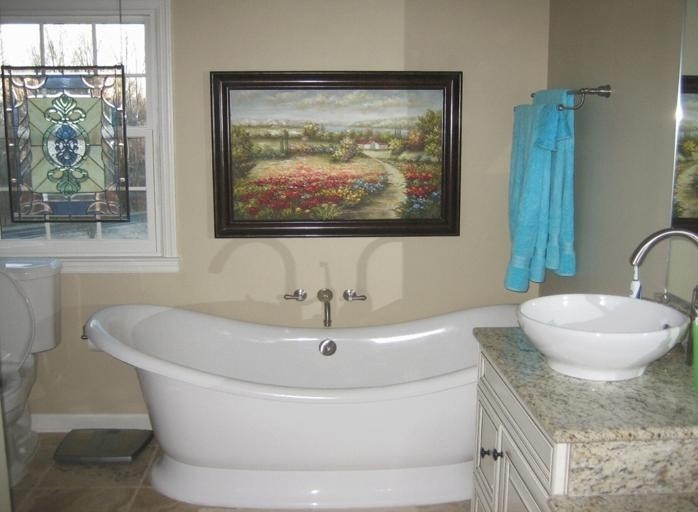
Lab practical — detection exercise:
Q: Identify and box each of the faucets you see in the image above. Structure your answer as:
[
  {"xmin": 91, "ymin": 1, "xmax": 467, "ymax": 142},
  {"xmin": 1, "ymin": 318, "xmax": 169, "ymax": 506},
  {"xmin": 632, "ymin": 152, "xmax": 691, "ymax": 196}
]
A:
[{"xmin": 630, "ymin": 226, "xmax": 698, "ymax": 267}]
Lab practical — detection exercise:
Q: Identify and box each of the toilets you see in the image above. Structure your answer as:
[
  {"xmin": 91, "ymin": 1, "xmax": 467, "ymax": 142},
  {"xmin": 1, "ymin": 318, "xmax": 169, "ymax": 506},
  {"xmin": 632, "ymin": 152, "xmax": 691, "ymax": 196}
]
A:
[{"xmin": 0, "ymin": 254, "xmax": 61, "ymax": 488}]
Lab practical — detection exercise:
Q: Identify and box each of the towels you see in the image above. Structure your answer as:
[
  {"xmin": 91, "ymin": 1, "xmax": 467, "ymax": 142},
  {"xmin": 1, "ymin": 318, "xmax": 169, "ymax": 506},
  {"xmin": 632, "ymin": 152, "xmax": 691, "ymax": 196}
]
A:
[
  {"xmin": 533, "ymin": 90, "xmax": 575, "ymax": 277},
  {"xmin": 503, "ymin": 105, "xmax": 571, "ymax": 292}
]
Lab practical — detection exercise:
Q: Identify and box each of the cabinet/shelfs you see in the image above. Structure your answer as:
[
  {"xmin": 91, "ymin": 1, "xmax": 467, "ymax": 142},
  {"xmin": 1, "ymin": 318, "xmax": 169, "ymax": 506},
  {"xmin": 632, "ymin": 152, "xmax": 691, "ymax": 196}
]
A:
[{"xmin": 470, "ymin": 343, "xmax": 570, "ymax": 512}]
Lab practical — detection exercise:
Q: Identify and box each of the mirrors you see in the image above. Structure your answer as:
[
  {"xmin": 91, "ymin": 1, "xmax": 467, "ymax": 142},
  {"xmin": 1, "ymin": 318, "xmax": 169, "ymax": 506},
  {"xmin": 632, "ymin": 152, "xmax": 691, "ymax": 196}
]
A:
[{"xmin": 671, "ymin": 0, "xmax": 698, "ymax": 235}]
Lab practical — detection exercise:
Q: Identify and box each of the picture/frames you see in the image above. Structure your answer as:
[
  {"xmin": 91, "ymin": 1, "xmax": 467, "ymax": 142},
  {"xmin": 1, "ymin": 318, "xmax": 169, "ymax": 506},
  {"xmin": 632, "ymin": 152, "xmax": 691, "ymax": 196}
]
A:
[
  {"xmin": 672, "ymin": 76, "xmax": 698, "ymax": 233},
  {"xmin": 210, "ymin": 71, "xmax": 460, "ymax": 237}
]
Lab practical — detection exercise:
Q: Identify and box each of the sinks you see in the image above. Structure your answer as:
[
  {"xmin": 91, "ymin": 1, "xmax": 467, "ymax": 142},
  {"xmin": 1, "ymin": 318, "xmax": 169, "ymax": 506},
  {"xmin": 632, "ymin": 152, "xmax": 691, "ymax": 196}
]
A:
[{"xmin": 516, "ymin": 292, "xmax": 691, "ymax": 382}]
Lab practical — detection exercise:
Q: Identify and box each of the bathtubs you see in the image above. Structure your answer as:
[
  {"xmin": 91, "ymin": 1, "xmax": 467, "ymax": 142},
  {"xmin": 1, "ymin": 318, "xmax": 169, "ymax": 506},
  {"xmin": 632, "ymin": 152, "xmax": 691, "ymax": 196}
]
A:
[{"xmin": 84, "ymin": 304, "xmax": 518, "ymax": 510}]
